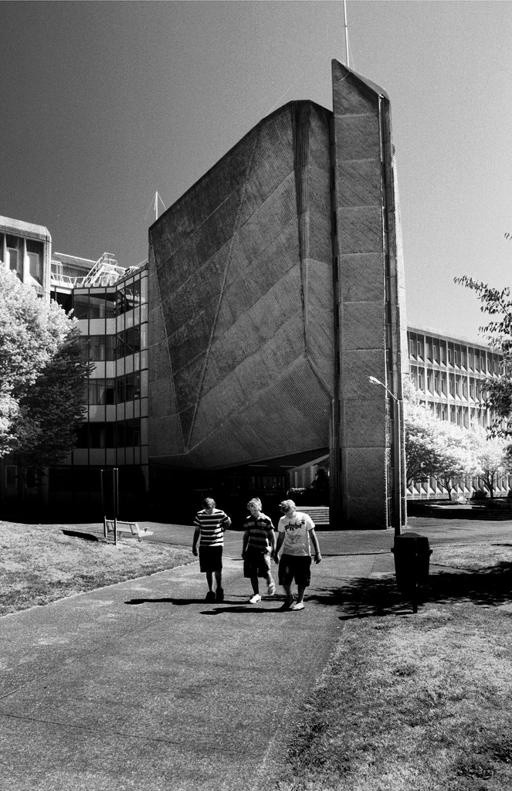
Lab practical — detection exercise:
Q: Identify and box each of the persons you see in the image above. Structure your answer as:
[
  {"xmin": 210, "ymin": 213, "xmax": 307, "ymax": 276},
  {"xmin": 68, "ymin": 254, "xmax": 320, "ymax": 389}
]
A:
[
  {"xmin": 189, "ymin": 497, "xmax": 232, "ymax": 603},
  {"xmin": 241, "ymin": 497, "xmax": 276, "ymax": 605},
  {"xmin": 273, "ymin": 498, "xmax": 322, "ymax": 612}
]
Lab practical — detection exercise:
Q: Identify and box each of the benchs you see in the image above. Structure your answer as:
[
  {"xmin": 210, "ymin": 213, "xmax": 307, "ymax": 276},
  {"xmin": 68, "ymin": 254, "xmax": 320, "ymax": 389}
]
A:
[
  {"xmin": 103, "ymin": 517, "xmax": 157, "ymax": 543},
  {"xmin": 288, "ymin": 506, "xmax": 333, "ymax": 532}
]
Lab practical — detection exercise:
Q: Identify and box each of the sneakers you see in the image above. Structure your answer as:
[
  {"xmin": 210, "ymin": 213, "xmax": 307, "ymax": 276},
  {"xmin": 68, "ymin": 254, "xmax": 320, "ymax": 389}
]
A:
[
  {"xmin": 266, "ymin": 578, "xmax": 276, "ymax": 596},
  {"xmin": 248, "ymin": 594, "xmax": 261, "ymax": 604},
  {"xmin": 291, "ymin": 603, "xmax": 305, "ymax": 611}
]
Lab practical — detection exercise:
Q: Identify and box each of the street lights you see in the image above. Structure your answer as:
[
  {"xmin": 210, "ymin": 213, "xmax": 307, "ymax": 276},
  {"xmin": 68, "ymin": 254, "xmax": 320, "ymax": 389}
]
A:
[{"xmin": 367, "ymin": 371, "xmax": 405, "ymax": 553}]
